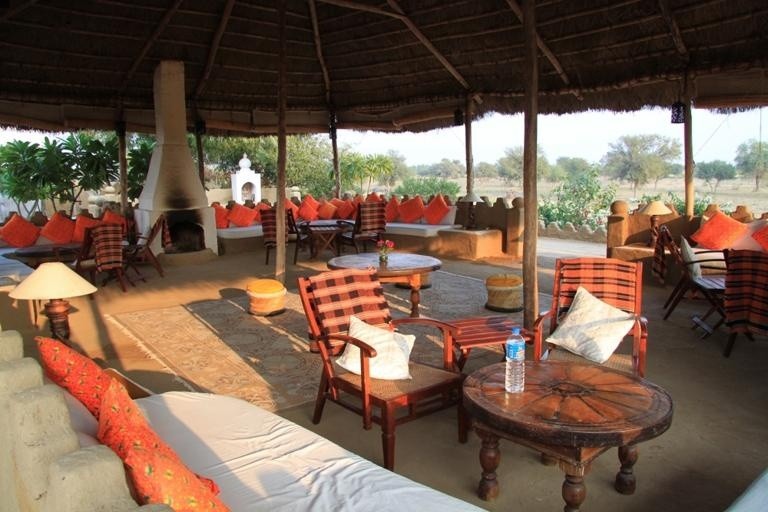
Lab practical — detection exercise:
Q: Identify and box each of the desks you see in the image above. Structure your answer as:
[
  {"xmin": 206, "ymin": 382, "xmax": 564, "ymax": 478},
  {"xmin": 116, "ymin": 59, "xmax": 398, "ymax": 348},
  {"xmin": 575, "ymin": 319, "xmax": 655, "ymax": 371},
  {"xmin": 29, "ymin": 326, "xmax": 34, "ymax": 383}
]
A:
[
  {"xmin": 462, "ymin": 358, "xmax": 672, "ymax": 512},
  {"xmin": 327, "ymin": 252, "xmax": 443, "ymax": 318}
]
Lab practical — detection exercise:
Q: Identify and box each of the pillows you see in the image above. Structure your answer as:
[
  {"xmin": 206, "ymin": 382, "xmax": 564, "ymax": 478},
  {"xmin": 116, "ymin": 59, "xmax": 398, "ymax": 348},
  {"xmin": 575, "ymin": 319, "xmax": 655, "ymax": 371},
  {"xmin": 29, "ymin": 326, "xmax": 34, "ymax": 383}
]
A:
[
  {"xmin": 690, "ymin": 213, "xmax": 768, "ymax": 251},
  {"xmin": 0, "ymin": 209, "xmax": 135, "ymax": 248},
  {"xmin": 34, "ymin": 337, "xmax": 230, "ymax": 512},
  {"xmin": 212, "ymin": 194, "xmax": 450, "ymax": 228}
]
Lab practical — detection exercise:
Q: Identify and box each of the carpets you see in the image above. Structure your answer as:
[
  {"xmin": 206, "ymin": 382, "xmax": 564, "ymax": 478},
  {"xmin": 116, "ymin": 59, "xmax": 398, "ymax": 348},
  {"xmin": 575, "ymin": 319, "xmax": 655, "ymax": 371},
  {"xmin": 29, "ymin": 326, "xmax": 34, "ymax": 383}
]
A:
[{"xmin": 104, "ymin": 271, "xmax": 552, "ymax": 415}]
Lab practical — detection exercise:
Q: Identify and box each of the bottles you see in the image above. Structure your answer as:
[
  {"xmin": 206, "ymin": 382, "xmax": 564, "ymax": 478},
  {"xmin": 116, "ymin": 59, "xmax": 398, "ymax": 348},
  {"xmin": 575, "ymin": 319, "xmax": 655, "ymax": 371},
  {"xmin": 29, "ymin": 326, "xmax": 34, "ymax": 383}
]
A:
[{"xmin": 504, "ymin": 327, "xmax": 525, "ymax": 394}]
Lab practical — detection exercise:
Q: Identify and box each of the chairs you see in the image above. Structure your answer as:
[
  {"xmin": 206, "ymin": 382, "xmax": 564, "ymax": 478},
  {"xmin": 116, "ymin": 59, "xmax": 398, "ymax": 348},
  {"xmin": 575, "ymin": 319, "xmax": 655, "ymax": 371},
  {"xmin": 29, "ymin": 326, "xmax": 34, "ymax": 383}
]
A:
[
  {"xmin": 71, "ymin": 214, "xmax": 164, "ymax": 292},
  {"xmin": 259, "ymin": 201, "xmax": 390, "ymax": 265},
  {"xmin": 658, "ymin": 226, "xmax": 768, "ymax": 358}
]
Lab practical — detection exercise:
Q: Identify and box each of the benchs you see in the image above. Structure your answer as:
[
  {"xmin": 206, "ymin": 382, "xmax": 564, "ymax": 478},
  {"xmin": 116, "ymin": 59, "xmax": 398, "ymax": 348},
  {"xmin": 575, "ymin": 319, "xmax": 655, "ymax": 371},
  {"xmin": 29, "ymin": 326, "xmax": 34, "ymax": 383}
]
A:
[
  {"xmin": 0, "ymin": 226, "xmax": 129, "ymax": 283},
  {"xmin": 58, "ymin": 391, "xmax": 489, "ymax": 512},
  {"xmin": 217, "ymin": 205, "xmax": 460, "ymax": 257}
]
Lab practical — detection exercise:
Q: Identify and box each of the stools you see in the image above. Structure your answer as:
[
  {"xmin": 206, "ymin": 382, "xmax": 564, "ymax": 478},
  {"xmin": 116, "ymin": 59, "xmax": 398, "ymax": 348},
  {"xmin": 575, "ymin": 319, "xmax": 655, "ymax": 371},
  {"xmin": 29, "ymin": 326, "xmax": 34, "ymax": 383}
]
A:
[{"xmin": 246, "ymin": 279, "xmax": 287, "ymax": 316}]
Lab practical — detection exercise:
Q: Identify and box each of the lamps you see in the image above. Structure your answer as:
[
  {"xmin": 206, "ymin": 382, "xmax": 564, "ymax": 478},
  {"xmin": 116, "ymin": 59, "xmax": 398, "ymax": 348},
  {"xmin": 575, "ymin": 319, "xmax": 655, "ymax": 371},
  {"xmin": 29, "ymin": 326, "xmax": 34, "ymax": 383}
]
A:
[
  {"xmin": 7, "ymin": 262, "xmax": 98, "ymax": 341},
  {"xmin": 640, "ymin": 201, "xmax": 673, "ymax": 247},
  {"xmin": 459, "ymin": 193, "xmax": 484, "ymax": 230}
]
